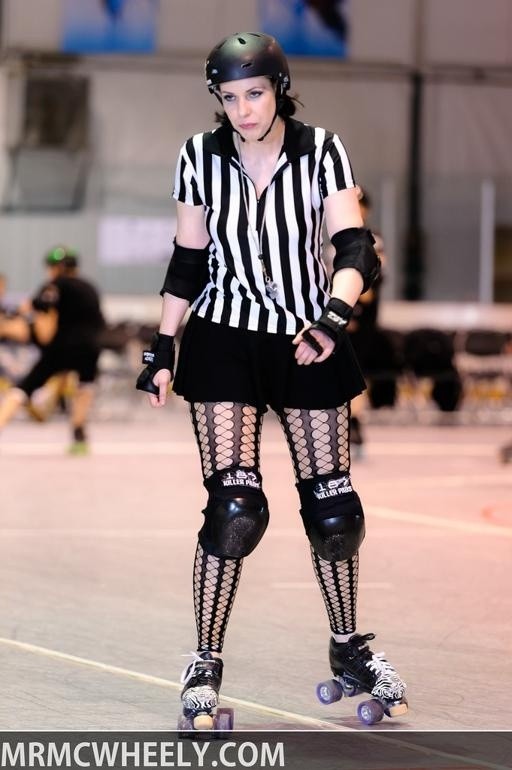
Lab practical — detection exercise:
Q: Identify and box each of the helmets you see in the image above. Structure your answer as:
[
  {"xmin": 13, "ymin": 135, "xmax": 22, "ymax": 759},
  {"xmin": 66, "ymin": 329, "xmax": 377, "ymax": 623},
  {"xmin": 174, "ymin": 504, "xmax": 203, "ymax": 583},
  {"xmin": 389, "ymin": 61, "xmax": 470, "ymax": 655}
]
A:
[{"xmin": 204, "ymin": 30, "xmax": 291, "ymax": 95}]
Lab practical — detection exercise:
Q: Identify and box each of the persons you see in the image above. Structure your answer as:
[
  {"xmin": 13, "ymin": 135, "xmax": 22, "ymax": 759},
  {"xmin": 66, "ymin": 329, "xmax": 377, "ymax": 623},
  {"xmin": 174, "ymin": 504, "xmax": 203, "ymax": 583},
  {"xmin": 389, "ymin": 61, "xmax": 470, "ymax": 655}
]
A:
[
  {"xmin": 323, "ymin": 185, "xmax": 387, "ymax": 447},
  {"xmin": 2, "ymin": 243, "xmax": 124, "ymax": 455},
  {"xmin": 133, "ymin": 31, "xmax": 409, "ymax": 717}
]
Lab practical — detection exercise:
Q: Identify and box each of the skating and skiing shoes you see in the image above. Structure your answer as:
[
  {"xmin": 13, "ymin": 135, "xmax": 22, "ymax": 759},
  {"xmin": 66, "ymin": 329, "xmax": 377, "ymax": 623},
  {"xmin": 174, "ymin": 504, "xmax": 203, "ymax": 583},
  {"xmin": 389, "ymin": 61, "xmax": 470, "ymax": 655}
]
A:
[
  {"xmin": 177, "ymin": 652, "xmax": 235, "ymax": 741},
  {"xmin": 316, "ymin": 632, "xmax": 409, "ymax": 726}
]
